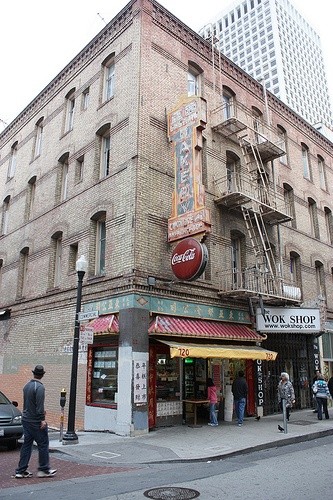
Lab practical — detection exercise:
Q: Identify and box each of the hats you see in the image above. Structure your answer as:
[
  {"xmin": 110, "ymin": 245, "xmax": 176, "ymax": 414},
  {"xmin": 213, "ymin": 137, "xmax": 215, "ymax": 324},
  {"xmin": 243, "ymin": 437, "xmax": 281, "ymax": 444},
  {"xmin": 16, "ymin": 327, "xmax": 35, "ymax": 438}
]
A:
[{"xmin": 31, "ymin": 365, "xmax": 46, "ymax": 375}]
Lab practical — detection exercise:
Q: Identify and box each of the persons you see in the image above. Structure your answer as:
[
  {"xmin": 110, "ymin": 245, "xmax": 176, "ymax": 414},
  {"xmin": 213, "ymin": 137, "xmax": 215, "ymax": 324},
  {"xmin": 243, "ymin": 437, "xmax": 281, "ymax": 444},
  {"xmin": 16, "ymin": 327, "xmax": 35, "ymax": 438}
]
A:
[
  {"xmin": 206, "ymin": 377, "xmax": 218, "ymax": 426},
  {"xmin": 14, "ymin": 365, "xmax": 57, "ymax": 478},
  {"xmin": 278, "ymin": 372, "xmax": 295, "ymax": 422},
  {"xmin": 231, "ymin": 370, "xmax": 248, "ymax": 426},
  {"xmin": 311, "ymin": 367, "xmax": 333, "ymax": 420}
]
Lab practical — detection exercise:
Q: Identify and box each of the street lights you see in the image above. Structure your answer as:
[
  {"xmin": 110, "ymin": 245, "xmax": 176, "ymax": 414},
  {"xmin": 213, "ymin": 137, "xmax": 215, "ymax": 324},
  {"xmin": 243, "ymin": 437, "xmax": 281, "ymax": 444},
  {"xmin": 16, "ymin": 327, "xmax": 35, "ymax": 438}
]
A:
[{"xmin": 62, "ymin": 253, "xmax": 88, "ymax": 440}]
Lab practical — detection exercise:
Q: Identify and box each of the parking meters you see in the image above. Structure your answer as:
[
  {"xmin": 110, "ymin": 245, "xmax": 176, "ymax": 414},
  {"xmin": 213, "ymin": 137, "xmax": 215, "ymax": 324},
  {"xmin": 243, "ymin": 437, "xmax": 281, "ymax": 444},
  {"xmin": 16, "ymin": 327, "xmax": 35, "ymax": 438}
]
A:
[{"xmin": 59, "ymin": 388, "xmax": 67, "ymax": 441}]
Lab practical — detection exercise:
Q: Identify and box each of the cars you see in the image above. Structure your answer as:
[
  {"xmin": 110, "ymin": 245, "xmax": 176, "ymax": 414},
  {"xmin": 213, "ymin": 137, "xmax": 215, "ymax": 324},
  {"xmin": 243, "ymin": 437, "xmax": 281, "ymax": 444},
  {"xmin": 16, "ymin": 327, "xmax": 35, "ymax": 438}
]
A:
[{"xmin": 0, "ymin": 391, "xmax": 24, "ymax": 450}]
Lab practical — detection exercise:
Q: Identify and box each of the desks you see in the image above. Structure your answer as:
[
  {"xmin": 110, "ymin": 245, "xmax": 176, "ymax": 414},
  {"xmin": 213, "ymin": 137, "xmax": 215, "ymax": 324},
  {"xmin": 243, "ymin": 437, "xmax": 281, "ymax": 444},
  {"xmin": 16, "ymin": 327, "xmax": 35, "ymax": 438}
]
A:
[{"xmin": 183, "ymin": 398, "xmax": 208, "ymax": 427}]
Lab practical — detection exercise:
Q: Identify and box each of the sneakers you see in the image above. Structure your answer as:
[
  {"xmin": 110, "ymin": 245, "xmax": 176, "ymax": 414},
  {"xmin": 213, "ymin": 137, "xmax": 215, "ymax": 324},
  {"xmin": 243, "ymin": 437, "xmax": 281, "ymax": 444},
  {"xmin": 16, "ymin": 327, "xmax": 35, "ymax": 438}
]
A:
[
  {"xmin": 14, "ymin": 469, "xmax": 33, "ymax": 478},
  {"xmin": 36, "ymin": 467, "xmax": 57, "ymax": 478}
]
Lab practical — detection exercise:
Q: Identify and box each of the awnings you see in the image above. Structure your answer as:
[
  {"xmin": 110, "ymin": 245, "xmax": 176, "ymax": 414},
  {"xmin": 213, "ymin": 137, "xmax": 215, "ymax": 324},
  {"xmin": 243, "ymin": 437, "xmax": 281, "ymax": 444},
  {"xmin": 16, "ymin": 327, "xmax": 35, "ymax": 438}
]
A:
[{"xmin": 156, "ymin": 339, "xmax": 278, "ymax": 361}]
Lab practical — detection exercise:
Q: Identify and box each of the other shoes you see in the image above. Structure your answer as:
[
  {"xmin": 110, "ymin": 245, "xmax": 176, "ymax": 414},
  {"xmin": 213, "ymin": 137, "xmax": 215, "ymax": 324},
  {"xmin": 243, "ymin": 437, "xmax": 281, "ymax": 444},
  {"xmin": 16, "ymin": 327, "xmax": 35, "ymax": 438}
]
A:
[
  {"xmin": 207, "ymin": 423, "xmax": 211, "ymax": 426},
  {"xmin": 312, "ymin": 410, "xmax": 318, "ymax": 413},
  {"xmin": 210, "ymin": 423, "xmax": 218, "ymax": 427}
]
